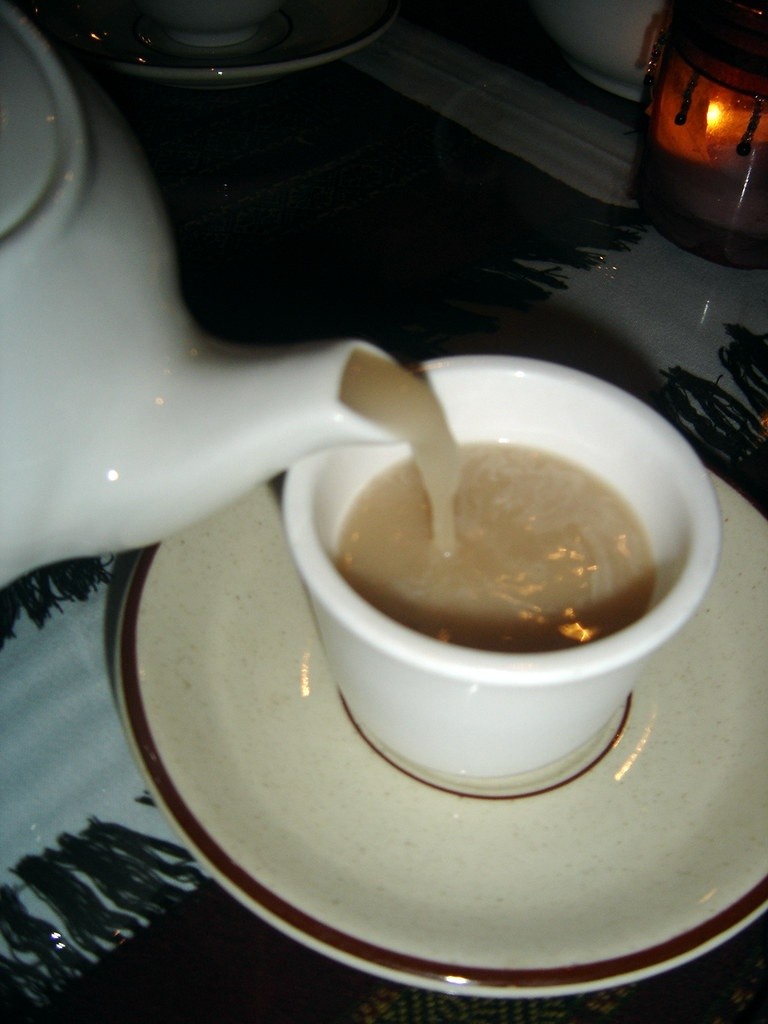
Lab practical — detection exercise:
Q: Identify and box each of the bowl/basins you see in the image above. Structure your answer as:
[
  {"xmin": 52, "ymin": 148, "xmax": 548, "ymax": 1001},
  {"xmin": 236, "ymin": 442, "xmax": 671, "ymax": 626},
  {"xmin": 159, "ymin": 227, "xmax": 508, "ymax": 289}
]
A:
[
  {"xmin": 137, "ymin": 0, "xmax": 295, "ymax": 49},
  {"xmin": 528, "ymin": 1, "xmax": 676, "ymax": 104}
]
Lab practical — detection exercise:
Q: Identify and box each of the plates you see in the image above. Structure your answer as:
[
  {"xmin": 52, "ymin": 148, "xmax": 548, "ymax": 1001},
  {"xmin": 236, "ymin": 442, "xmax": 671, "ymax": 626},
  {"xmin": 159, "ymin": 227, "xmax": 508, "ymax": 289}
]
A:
[
  {"xmin": 74, "ymin": 1, "xmax": 412, "ymax": 88},
  {"xmin": 105, "ymin": 384, "xmax": 767, "ymax": 1000}
]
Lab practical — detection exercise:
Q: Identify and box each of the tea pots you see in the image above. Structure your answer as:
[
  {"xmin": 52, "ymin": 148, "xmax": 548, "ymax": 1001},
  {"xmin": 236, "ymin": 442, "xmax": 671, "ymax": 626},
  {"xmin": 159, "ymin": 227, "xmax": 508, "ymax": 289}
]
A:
[{"xmin": 1, "ymin": 3, "xmax": 430, "ymax": 594}]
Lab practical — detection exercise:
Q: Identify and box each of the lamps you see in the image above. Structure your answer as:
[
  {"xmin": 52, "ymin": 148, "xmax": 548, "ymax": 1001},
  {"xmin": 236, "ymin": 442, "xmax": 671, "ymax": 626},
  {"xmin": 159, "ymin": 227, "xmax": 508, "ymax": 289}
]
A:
[{"xmin": 636, "ymin": 0, "xmax": 768, "ymax": 270}]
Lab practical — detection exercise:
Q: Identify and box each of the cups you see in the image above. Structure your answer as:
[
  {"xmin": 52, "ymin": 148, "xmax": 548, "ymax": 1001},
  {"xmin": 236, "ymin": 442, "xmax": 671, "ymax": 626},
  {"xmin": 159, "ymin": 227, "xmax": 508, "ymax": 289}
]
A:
[{"xmin": 283, "ymin": 358, "xmax": 725, "ymax": 781}]
